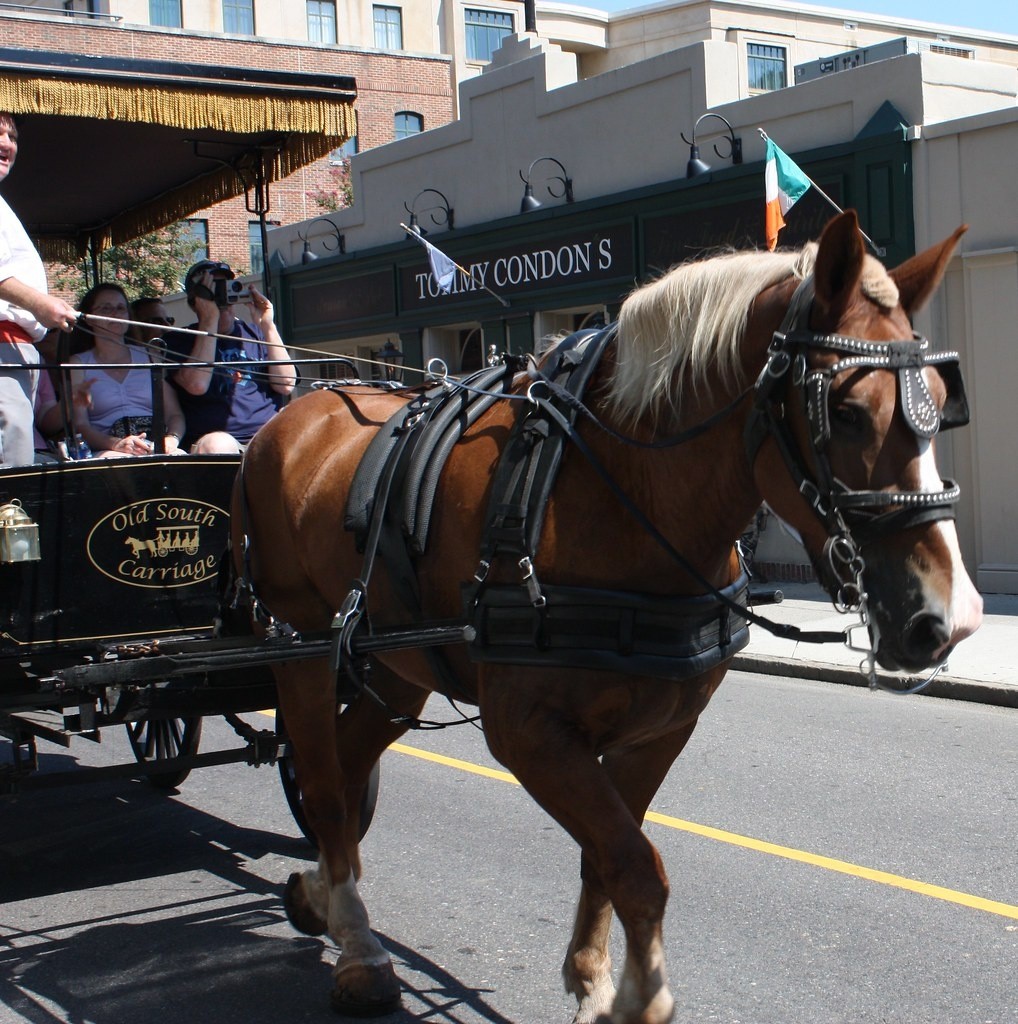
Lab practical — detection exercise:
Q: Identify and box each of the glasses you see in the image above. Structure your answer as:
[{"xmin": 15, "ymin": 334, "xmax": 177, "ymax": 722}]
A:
[
  {"xmin": 91, "ymin": 303, "xmax": 128, "ymax": 315},
  {"xmin": 190, "ymin": 261, "xmax": 230, "ymax": 279},
  {"xmin": 141, "ymin": 317, "xmax": 175, "ymax": 326}
]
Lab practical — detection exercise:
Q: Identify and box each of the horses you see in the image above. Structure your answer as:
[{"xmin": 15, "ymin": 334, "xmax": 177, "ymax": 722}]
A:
[{"xmin": 226, "ymin": 207, "xmax": 991, "ymax": 1023}]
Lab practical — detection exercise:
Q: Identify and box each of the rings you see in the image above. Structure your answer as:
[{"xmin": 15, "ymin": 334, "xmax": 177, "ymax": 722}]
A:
[{"xmin": 261, "ymin": 301, "xmax": 264, "ymax": 303}]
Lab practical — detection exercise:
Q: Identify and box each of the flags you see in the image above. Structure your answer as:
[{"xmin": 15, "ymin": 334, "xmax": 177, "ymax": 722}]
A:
[
  {"xmin": 409, "ymin": 226, "xmax": 458, "ymax": 294},
  {"xmin": 765, "ymin": 138, "xmax": 811, "ymax": 253}
]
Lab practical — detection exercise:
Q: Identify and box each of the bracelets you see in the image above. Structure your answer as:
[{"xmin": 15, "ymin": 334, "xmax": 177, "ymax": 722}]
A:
[
  {"xmin": 164, "ymin": 432, "xmax": 180, "ymax": 440},
  {"xmin": 111, "ymin": 438, "xmax": 122, "ymax": 451}
]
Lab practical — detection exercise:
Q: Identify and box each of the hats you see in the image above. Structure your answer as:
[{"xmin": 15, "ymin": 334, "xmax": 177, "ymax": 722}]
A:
[{"xmin": 185, "ymin": 260, "xmax": 235, "ymax": 295}]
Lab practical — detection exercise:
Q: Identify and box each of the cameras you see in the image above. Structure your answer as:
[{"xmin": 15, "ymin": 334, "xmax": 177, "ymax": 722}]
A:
[{"xmin": 212, "ymin": 278, "xmax": 253, "ymax": 308}]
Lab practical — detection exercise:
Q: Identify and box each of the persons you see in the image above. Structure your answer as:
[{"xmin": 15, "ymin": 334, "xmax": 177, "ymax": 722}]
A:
[
  {"xmin": 130, "ymin": 298, "xmax": 176, "ymax": 343},
  {"xmin": 0, "ymin": 111, "xmax": 77, "ymax": 468},
  {"xmin": 159, "ymin": 259, "xmax": 301, "ymax": 455},
  {"xmin": 63, "ymin": 283, "xmax": 187, "ymax": 458},
  {"xmin": 34, "ymin": 328, "xmax": 70, "ymax": 400},
  {"xmin": 33, "ymin": 353, "xmax": 98, "ymax": 464}
]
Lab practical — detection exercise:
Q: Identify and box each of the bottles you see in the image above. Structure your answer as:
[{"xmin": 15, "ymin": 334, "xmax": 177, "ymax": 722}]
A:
[{"xmin": 76, "ymin": 433, "xmax": 93, "ymax": 459}]
